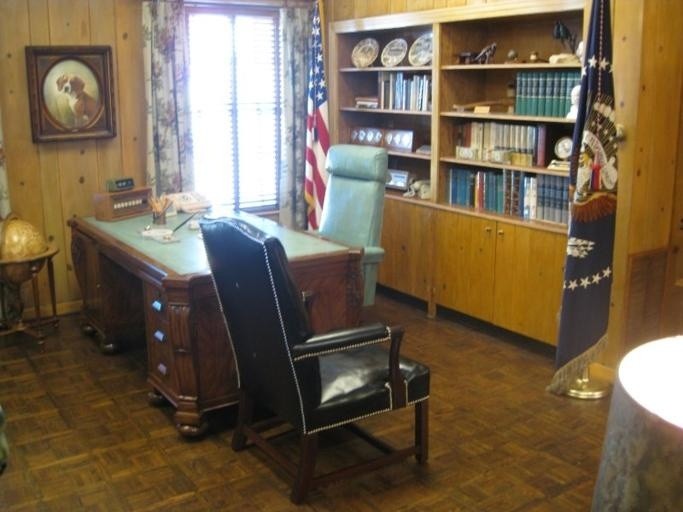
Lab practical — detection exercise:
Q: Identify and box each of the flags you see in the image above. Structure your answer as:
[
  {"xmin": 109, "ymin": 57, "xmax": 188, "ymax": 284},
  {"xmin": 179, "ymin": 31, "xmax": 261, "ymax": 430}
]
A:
[
  {"xmin": 303, "ymin": 0, "xmax": 330, "ymax": 233},
  {"xmin": 544, "ymin": 0, "xmax": 619, "ymax": 393}
]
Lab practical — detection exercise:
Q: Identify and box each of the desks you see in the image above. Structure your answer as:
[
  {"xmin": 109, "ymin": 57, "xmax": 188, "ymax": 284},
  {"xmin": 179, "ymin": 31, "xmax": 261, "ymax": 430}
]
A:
[
  {"xmin": 591, "ymin": 335, "xmax": 683, "ymax": 512},
  {"xmin": 66, "ymin": 205, "xmax": 365, "ymax": 440}
]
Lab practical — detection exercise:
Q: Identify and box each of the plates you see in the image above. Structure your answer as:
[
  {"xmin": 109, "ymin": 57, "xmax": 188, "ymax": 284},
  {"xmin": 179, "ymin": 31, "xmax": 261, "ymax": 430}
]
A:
[
  {"xmin": 407, "ymin": 32, "xmax": 433, "ymax": 67},
  {"xmin": 141, "ymin": 229, "xmax": 173, "ymax": 238},
  {"xmin": 350, "ymin": 38, "xmax": 379, "ymax": 68},
  {"xmin": 380, "ymin": 39, "xmax": 408, "ymax": 67}
]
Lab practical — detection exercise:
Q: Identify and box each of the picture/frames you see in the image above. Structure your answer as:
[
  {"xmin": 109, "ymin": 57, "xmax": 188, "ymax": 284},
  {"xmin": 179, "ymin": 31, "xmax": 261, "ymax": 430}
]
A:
[{"xmin": 25, "ymin": 45, "xmax": 117, "ymax": 144}]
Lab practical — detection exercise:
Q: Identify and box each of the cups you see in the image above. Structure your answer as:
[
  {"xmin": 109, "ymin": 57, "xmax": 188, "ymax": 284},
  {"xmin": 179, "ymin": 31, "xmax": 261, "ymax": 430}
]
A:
[{"xmin": 152, "ymin": 212, "xmax": 167, "ymax": 226}]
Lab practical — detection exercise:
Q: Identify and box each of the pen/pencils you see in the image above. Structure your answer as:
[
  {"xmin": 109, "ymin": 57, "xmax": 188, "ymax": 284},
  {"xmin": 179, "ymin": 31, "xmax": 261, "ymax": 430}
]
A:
[
  {"xmin": 173, "ymin": 211, "xmax": 197, "ymax": 232},
  {"xmin": 148, "ymin": 192, "xmax": 173, "ymax": 213}
]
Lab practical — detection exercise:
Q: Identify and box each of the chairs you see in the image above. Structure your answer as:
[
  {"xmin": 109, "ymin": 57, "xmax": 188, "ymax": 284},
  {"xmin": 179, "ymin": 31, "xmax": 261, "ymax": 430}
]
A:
[
  {"xmin": 303, "ymin": 144, "xmax": 389, "ymax": 306},
  {"xmin": 198, "ymin": 215, "xmax": 431, "ymax": 505}
]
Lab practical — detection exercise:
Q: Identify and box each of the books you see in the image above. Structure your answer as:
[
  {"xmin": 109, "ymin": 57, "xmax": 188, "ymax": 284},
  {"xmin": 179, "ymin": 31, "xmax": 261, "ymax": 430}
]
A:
[
  {"xmin": 449, "ymin": 119, "xmax": 569, "ymax": 226},
  {"xmin": 377, "ymin": 70, "xmax": 432, "ymax": 112},
  {"xmin": 515, "ymin": 70, "xmax": 581, "ymax": 118}
]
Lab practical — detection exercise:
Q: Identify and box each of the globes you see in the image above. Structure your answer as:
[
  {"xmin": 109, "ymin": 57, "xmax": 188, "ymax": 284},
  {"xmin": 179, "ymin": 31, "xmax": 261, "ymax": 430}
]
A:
[{"xmin": 0, "ymin": 211, "xmax": 60, "ymax": 354}]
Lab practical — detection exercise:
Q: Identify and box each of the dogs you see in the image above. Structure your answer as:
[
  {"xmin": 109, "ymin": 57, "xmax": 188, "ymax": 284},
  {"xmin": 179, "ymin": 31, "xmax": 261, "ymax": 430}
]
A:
[{"xmin": 55, "ymin": 73, "xmax": 101, "ymax": 126}]
